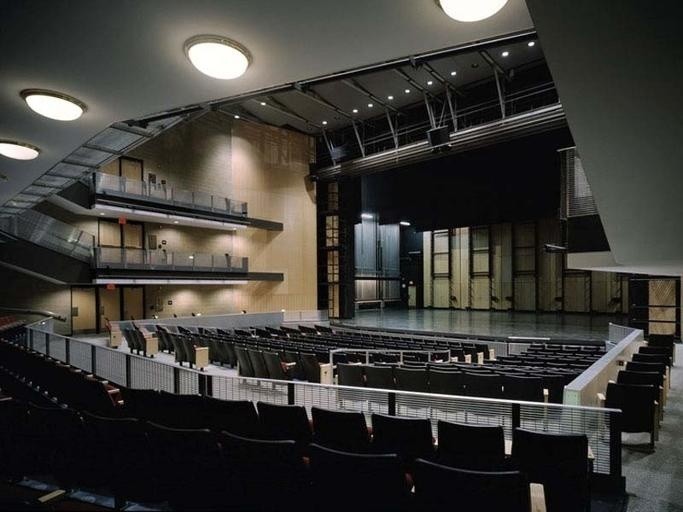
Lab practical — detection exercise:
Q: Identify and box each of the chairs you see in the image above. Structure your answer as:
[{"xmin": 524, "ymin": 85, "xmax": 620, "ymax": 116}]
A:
[
  {"xmin": 71, "ymin": 381, "xmax": 631, "ymax": 512},
  {"xmin": 336, "ymin": 344, "xmax": 609, "ymax": 435},
  {"xmin": 1, "ymin": 338, "xmax": 123, "ymax": 493},
  {"xmin": 105, "ymin": 313, "xmax": 491, "ymax": 383},
  {"xmin": 604, "ymin": 332, "xmax": 675, "ymax": 454},
  {"xmin": 234, "ymin": 346, "xmax": 296, "ymax": 395}
]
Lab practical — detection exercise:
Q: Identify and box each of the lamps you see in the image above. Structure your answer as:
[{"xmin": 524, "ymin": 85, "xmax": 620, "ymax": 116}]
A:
[
  {"xmin": 181, "ymin": 34, "xmax": 254, "ymax": 83},
  {"xmin": 19, "ymin": 87, "xmax": 89, "ymax": 123},
  {"xmin": 0, "ymin": 139, "xmax": 41, "ymax": 162}
]
